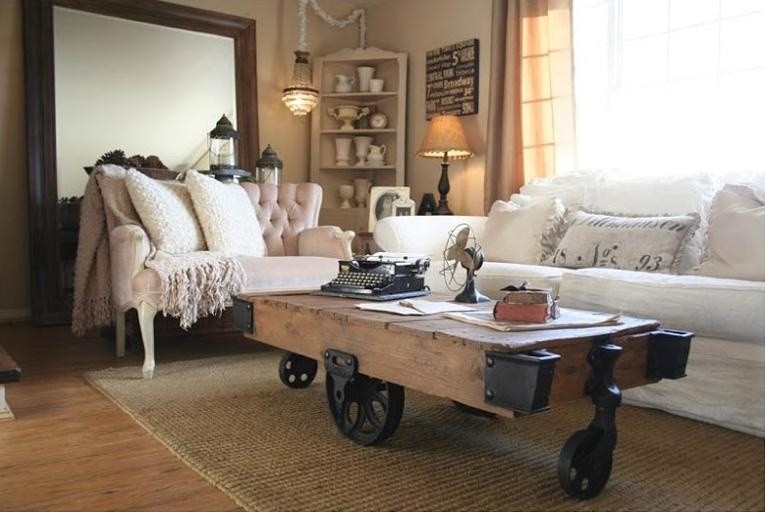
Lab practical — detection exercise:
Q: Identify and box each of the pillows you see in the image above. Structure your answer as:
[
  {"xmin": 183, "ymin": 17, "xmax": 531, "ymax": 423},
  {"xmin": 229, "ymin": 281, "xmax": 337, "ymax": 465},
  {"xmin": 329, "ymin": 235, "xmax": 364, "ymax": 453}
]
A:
[
  {"xmin": 186, "ymin": 169, "xmax": 268, "ymax": 256},
  {"xmin": 482, "ymin": 177, "xmax": 765, "ymax": 285},
  {"xmin": 125, "ymin": 167, "xmax": 208, "ymax": 254}
]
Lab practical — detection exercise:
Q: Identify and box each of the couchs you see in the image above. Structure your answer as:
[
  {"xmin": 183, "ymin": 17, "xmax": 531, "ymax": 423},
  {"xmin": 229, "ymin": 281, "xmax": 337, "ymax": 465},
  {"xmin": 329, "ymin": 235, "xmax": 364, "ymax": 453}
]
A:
[{"xmin": 370, "ymin": 176, "xmax": 765, "ymax": 443}]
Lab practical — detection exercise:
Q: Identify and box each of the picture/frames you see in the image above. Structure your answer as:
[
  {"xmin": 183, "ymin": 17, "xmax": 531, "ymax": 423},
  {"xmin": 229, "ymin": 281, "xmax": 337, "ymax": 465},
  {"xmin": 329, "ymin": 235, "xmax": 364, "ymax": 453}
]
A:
[
  {"xmin": 392, "ymin": 200, "xmax": 414, "ymax": 218},
  {"xmin": 369, "ymin": 186, "xmax": 410, "ymax": 232}
]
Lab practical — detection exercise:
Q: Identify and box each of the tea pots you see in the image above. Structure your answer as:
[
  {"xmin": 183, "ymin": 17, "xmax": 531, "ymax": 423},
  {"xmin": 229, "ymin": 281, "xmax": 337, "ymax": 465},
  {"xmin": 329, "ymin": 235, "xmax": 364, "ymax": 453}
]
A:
[
  {"xmin": 367, "ymin": 145, "xmax": 387, "ymax": 162},
  {"xmin": 333, "ymin": 73, "xmax": 355, "ymax": 93}
]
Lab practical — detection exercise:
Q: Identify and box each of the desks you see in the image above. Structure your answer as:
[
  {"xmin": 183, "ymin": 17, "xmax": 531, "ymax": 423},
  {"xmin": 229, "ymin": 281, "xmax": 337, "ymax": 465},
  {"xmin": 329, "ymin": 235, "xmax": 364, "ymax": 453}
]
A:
[{"xmin": 356, "ymin": 232, "xmax": 384, "ymax": 258}]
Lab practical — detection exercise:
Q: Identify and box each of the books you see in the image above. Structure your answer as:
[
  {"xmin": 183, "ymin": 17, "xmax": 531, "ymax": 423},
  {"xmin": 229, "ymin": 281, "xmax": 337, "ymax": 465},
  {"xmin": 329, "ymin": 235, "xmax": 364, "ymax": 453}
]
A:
[
  {"xmin": 493, "ymin": 300, "xmax": 564, "ymax": 323},
  {"xmin": 504, "ymin": 290, "xmax": 554, "ymax": 305},
  {"xmin": 444, "ymin": 306, "xmax": 625, "ymax": 333}
]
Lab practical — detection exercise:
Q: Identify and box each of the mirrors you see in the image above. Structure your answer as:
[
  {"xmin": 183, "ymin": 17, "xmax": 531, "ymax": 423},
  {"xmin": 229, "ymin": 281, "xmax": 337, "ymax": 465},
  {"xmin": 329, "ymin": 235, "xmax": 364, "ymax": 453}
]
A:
[{"xmin": 20, "ymin": 0, "xmax": 260, "ymax": 328}]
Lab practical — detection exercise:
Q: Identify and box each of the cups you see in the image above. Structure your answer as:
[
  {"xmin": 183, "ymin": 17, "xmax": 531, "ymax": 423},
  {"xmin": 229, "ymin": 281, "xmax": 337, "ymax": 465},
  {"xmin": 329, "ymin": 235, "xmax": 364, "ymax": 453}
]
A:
[
  {"xmin": 369, "ymin": 79, "xmax": 385, "ymax": 93},
  {"xmin": 356, "ymin": 67, "xmax": 376, "ymax": 93}
]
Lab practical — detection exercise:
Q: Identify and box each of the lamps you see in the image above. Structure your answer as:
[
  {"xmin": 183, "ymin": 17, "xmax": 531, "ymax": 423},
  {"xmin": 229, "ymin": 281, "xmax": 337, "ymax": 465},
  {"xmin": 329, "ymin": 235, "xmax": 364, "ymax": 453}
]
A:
[
  {"xmin": 281, "ymin": 0, "xmax": 321, "ymax": 118},
  {"xmin": 206, "ymin": 111, "xmax": 241, "ymax": 170},
  {"xmin": 256, "ymin": 144, "xmax": 284, "ymax": 186},
  {"xmin": 415, "ymin": 114, "xmax": 475, "ymax": 216}
]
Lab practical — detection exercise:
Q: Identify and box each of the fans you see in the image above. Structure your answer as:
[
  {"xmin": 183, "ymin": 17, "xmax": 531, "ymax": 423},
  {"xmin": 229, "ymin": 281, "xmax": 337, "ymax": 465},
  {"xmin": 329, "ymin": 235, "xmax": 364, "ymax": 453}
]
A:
[{"xmin": 441, "ymin": 220, "xmax": 486, "ymax": 302}]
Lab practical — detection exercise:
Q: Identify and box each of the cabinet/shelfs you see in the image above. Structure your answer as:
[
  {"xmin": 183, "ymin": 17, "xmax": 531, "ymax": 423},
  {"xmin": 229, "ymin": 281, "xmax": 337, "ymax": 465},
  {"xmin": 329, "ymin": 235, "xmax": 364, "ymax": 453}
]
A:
[{"xmin": 308, "ymin": 45, "xmax": 409, "ymax": 227}]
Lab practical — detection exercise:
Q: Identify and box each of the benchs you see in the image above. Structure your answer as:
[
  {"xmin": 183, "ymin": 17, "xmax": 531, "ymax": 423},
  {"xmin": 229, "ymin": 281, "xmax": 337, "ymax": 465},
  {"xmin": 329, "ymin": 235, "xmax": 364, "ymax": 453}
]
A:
[{"xmin": 71, "ymin": 163, "xmax": 356, "ymax": 381}]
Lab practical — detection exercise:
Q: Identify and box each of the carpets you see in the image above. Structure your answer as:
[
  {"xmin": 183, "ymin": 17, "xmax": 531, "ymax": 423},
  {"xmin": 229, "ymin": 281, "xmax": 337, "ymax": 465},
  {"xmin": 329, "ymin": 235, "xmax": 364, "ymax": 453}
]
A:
[{"xmin": 81, "ymin": 349, "xmax": 764, "ymax": 512}]
